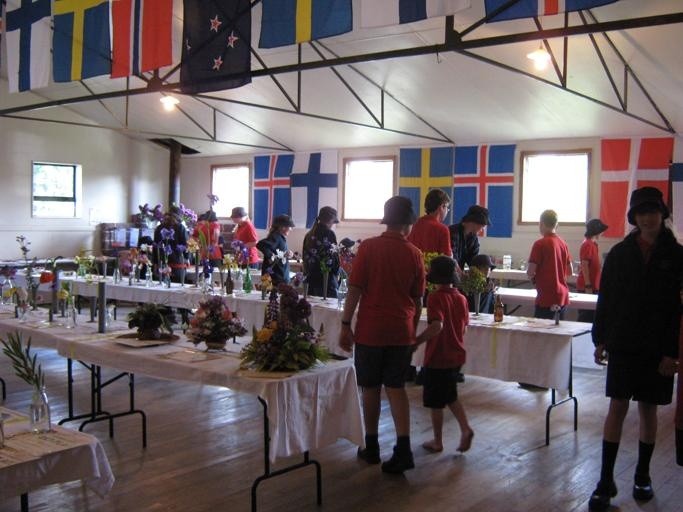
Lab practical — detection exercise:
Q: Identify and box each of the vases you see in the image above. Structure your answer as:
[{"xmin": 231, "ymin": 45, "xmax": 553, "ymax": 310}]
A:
[
  {"xmin": 164, "ymin": 263, "xmax": 171, "ymax": 288},
  {"xmin": 204, "ymin": 259, "xmax": 212, "ymax": 287},
  {"xmin": 2, "ymin": 276, "xmax": 13, "ymax": 314},
  {"xmin": 145, "ymin": 268, "xmax": 153, "ymax": 288},
  {"xmin": 129, "ymin": 271, "xmax": 136, "ymax": 286},
  {"xmin": 225, "ymin": 271, "xmax": 235, "ymax": 294},
  {"xmin": 113, "ymin": 268, "xmax": 121, "ymax": 285},
  {"xmin": 555, "ymin": 315, "xmax": 560, "ymax": 325},
  {"xmin": 67, "ymin": 296, "xmax": 78, "ymax": 328},
  {"xmin": 321, "ymin": 271, "xmax": 329, "ymax": 301},
  {"xmin": 494, "ymin": 294, "xmax": 504, "ymax": 322},
  {"xmin": 243, "ymin": 257, "xmax": 254, "ymax": 293},
  {"xmin": 205, "ymin": 342, "xmax": 228, "ymax": 353},
  {"xmin": 262, "ymin": 289, "xmax": 268, "ymax": 300},
  {"xmin": 337, "ymin": 279, "xmax": 348, "ymax": 308},
  {"xmin": 473, "ymin": 293, "xmax": 481, "ymax": 316},
  {"xmin": 181, "ymin": 269, "xmax": 185, "ymax": 287}
]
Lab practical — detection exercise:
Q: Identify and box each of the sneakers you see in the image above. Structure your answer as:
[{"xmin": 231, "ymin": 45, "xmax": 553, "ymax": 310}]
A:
[
  {"xmin": 382, "ymin": 443, "xmax": 415, "ymax": 475},
  {"xmin": 357, "ymin": 442, "xmax": 380, "ymax": 465}
]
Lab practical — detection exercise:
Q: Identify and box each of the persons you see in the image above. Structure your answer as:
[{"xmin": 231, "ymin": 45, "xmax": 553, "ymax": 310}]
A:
[
  {"xmin": 339, "ymin": 196, "xmax": 425, "ymax": 476},
  {"xmin": 194, "ymin": 214, "xmax": 207, "ymax": 240},
  {"xmin": 589, "ymin": 186, "xmax": 683, "ymax": 512},
  {"xmin": 461, "ymin": 255, "xmax": 496, "ymax": 314},
  {"xmin": 256, "ymin": 214, "xmax": 296, "ymax": 288},
  {"xmin": 527, "ymin": 210, "xmax": 574, "ymax": 320},
  {"xmin": 302, "ymin": 206, "xmax": 341, "ymax": 299},
  {"xmin": 577, "ymin": 219, "xmax": 608, "ymax": 321},
  {"xmin": 448, "ymin": 205, "xmax": 491, "ymax": 272},
  {"xmin": 416, "ymin": 256, "xmax": 474, "ymax": 452},
  {"xmin": 136, "ymin": 236, "xmax": 153, "ymax": 279},
  {"xmin": 198, "ymin": 211, "xmax": 225, "ymax": 267},
  {"xmin": 230, "ymin": 207, "xmax": 260, "ymax": 269},
  {"xmin": 407, "ymin": 189, "xmax": 453, "ymax": 307},
  {"xmin": 339, "ymin": 238, "xmax": 355, "ymax": 256}
]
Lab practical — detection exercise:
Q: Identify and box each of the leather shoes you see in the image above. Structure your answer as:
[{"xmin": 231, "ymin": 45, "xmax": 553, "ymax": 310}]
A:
[
  {"xmin": 631, "ymin": 474, "xmax": 655, "ymax": 501},
  {"xmin": 588, "ymin": 479, "xmax": 619, "ymax": 508}
]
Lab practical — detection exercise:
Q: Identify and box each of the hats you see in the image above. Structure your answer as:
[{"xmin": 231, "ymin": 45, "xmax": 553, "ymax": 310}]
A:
[
  {"xmin": 272, "ymin": 213, "xmax": 296, "ymax": 227},
  {"xmin": 339, "ymin": 238, "xmax": 355, "ymax": 248},
  {"xmin": 583, "ymin": 218, "xmax": 607, "ymax": 237},
  {"xmin": 207, "ymin": 210, "xmax": 217, "ymax": 221},
  {"xmin": 317, "ymin": 206, "xmax": 339, "ymax": 223},
  {"xmin": 381, "ymin": 196, "xmax": 417, "ymax": 225},
  {"xmin": 230, "ymin": 207, "xmax": 247, "ymax": 218},
  {"xmin": 474, "ymin": 254, "xmax": 494, "ymax": 271},
  {"xmin": 198, "ymin": 214, "xmax": 208, "ymax": 220},
  {"xmin": 426, "ymin": 255, "xmax": 459, "ymax": 284},
  {"xmin": 626, "ymin": 186, "xmax": 670, "ymax": 226},
  {"xmin": 460, "ymin": 204, "xmax": 491, "ymax": 226}
]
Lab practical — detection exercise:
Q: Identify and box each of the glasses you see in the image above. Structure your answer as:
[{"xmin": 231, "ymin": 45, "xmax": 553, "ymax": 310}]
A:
[{"xmin": 444, "ymin": 204, "xmax": 451, "ymax": 211}]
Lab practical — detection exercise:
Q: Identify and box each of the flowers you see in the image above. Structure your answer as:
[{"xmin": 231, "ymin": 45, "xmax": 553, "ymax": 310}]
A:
[
  {"xmin": 185, "ymin": 295, "xmax": 248, "ymax": 347},
  {"xmin": 58, "ymin": 280, "xmax": 73, "ymax": 304},
  {"xmin": 113, "ymin": 250, "xmax": 125, "ymax": 268},
  {"xmin": 230, "ymin": 240, "xmax": 249, "ymax": 262},
  {"xmin": 259, "ymin": 272, "xmax": 274, "ymax": 290},
  {"xmin": 137, "ymin": 254, "xmax": 153, "ymax": 267},
  {"xmin": 311, "ymin": 235, "xmax": 340, "ymax": 272},
  {"xmin": 137, "ymin": 202, "xmax": 198, "ymax": 264},
  {"xmin": 22, "ymin": 265, "xmax": 55, "ymax": 311},
  {"xmin": 337, "ymin": 255, "xmax": 350, "ymax": 279},
  {"xmin": 16, "ymin": 234, "xmax": 31, "ymax": 267},
  {"xmin": 239, "ymin": 284, "xmax": 336, "ymax": 373},
  {"xmin": 462, "ymin": 266, "xmax": 485, "ymax": 293},
  {"xmin": 220, "ymin": 253, "xmax": 239, "ymax": 272},
  {"xmin": 175, "ymin": 238, "xmax": 200, "ymax": 269},
  {"xmin": 199, "ymin": 193, "xmax": 219, "ymax": 259},
  {"xmin": 127, "ymin": 247, "xmax": 137, "ymax": 272},
  {"xmin": 549, "ymin": 303, "xmax": 568, "ymax": 314}
]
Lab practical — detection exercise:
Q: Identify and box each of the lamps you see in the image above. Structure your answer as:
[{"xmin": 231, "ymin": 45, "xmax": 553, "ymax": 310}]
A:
[
  {"xmin": 526, "ymin": 16, "xmax": 551, "ymax": 70},
  {"xmin": 159, "ymin": 91, "xmax": 179, "ymax": 113}
]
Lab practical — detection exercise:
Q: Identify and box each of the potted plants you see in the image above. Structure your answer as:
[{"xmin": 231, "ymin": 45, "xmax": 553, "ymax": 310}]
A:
[
  {"xmin": 127, "ymin": 297, "xmax": 195, "ymax": 340},
  {"xmin": 0, "ymin": 329, "xmax": 51, "ymax": 435}
]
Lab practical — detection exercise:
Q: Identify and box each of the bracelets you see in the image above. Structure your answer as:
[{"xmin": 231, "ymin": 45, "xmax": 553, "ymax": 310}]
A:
[
  {"xmin": 585, "ymin": 284, "xmax": 592, "ymax": 288},
  {"xmin": 341, "ymin": 321, "xmax": 351, "ymax": 325}
]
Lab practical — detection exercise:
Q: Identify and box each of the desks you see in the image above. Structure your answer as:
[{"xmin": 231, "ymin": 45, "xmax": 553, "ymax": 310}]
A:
[
  {"xmin": 186, "ymin": 266, "xmax": 298, "ymax": 286},
  {"xmin": 493, "ymin": 285, "xmax": 598, "ymax": 315},
  {"xmin": 464, "ymin": 267, "xmax": 578, "ymax": 288},
  {"xmin": 1, "ymin": 405, "xmax": 99, "ymax": 512},
  {"xmin": 0, "ymin": 302, "xmax": 129, "ymax": 439},
  {"xmin": 2, "ymin": 266, "xmax": 595, "ymax": 446},
  {"xmin": 57, "ymin": 327, "xmax": 355, "ymax": 512}
]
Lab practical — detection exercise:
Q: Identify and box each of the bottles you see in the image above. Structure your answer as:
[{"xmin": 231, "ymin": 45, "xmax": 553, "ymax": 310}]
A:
[
  {"xmin": 337, "ymin": 278, "xmax": 348, "ymax": 311},
  {"xmin": 2, "ymin": 276, "xmax": 13, "ymax": 305},
  {"xmin": 164, "ymin": 275, "xmax": 171, "ymax": 288},
  {"xmin": 29, "ymin": 385, "xmax": 51, "ymax": 434},
  {"xmin": 146, "ymin": 267, "xmax": 153, "ymax": 288},
  {"xmin": 245, "ymin": 266, "xmax": 252, "ymax": 293},
  {"xmin": 225, "ymin": 269, "xmax": 233, "ymax": 294},
  {"xmin": 494, "ymin": 294, "xmax": 504, "ymax": 322},
  {"xmin": 112, "ymin": 267, "xmax": 120, "ymax": 285},
  {"xmin": 63, "ymin": 295, "xmax": 78, "ymax": 329}
]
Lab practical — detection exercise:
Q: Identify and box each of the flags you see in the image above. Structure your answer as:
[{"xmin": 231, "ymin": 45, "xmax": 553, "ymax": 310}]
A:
[
  {"xmin": 600, "ymin": 139, "xmax": 672, "ymax": 238},
  {"xmin": 112, "ymin": 0, "xmax": 173, "ymax": 78},
  {"xmin": 254, "ymin": 156, "xmax": 293, "ymax": 228},
  {"xmin": 259, "ymin": 0, "xmax": 353, "ymax": 48},
  {"xmin": 453, "ymin": 145, "xmax": 515, "ymax": 238},
  {"xmin": 180, "ymin": 0, "xmax": 251, "ymax": 94},
  {"xmin": 672, "ymin": 139, "xmax": 683, "ymax": 246},
  {"xmin": 484, "ymin": 0, "xmax": 617, "ymax": 23},
  {"xmin": 291, "ymin": 151, "xmax": 338, "ymax": 228},
  {"xmin": 53, "ymin": 0, "xmax": 111, "ymax": 82},
  {"xmin": 5, "ymin": 0, "xmax": 51, "ymax": 92},
  {"xmin": 362, "ymin": 0, "xmax": 471, "ymax": 27},
  {"xmin": 399, "ymin": 148, "xmax": 452, "ymax": 227}
]
